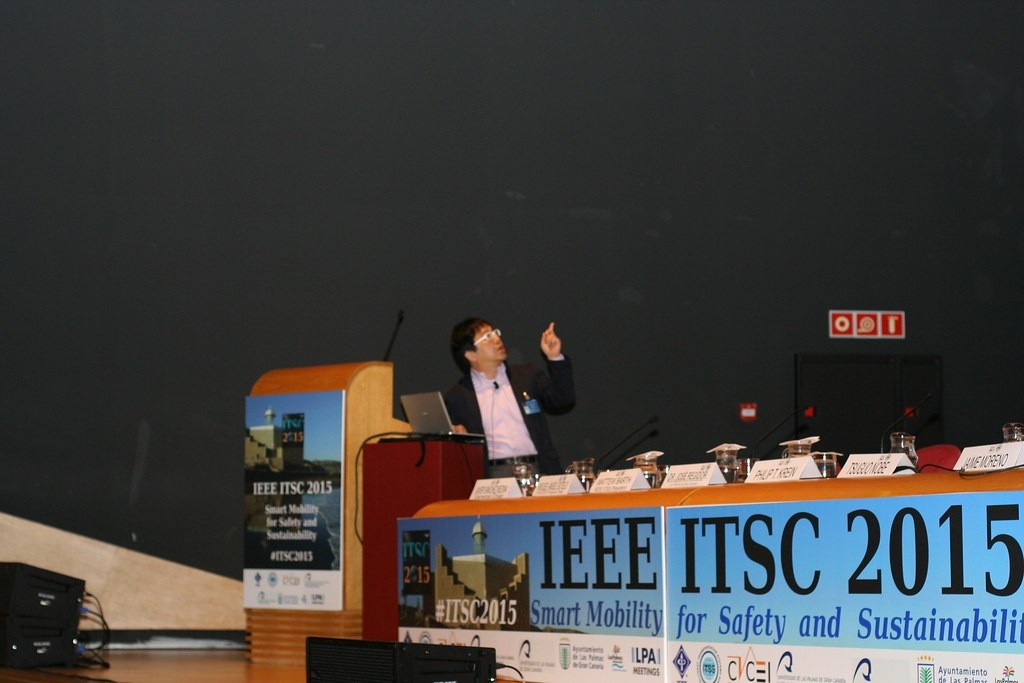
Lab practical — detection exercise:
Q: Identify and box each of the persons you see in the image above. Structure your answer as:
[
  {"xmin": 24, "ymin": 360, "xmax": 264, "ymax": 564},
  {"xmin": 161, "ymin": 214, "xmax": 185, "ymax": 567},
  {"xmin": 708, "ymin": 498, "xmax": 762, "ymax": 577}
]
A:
[{"xmin": 444, "ymin": 316, "xmax": 578, "ymax": 480}]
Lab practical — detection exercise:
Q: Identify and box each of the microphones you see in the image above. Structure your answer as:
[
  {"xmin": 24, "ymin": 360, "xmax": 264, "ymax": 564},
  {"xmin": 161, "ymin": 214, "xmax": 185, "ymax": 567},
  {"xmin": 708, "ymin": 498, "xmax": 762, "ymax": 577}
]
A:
[
  {"xmin": 881, "ymin": 391, "xmax": 935, "ymax": 453},
  {"xmin": 383, "ymin": 309, "xmax": 405, "ymax": 361},
  {"xmin": 596, "ymin": 415, "xmax": 658, "ymax": 461},
  {"xmin": 746, "ymin": 403, "xmax": 809, "ymax": 451},
  {"xmin": 493, "ymin": 381, "xmax": 499, "ymax": 389}
]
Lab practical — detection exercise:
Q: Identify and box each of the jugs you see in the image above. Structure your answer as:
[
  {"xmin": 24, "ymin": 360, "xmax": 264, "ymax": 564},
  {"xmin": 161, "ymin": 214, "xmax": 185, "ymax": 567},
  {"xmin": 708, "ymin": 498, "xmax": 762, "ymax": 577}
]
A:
[
  {"xmin": 890, "ymin": 432, "xmax": 918, "ymax": 468},
  {"xmin": 715, "ymin": 449, "xmax": 737, "ymax": 483},
  {"xmin": 782, "ymin": 440, "xmax": 811, "ymax": 459},
  {"xmin": 1002, "ymin": 423, "xmax": 1024, "ymax": 442},
  {"xmin": 633, "ymin": 456, "xmax": 657, "ymax": 488},
  {"xmin": 512, "ymin": 463, "xmax": 534, "ymax": 496},
  {"xmin": 565, "ymin": 460, "xmax": 594, "ymax": 493}
]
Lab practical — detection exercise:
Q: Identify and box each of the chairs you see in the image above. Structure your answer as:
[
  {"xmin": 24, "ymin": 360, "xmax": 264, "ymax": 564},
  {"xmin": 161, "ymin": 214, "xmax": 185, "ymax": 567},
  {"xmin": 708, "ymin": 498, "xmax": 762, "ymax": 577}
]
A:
[{"xmin": 916, "ymin": 443, "xmax": 961, "ymax": 472}]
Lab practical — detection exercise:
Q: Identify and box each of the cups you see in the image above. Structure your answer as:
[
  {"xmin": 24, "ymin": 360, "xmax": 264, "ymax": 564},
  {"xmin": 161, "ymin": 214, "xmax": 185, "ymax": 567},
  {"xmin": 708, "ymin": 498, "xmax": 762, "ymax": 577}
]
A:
[
  {"xmin": 535, "ymin": 474, "xmax": 546, "ymax": 487},
  {"xmin": 734, "ymin": 459, "xmax": 751, "ymax": 482},
  {"xmin": 655, "ymin": 465, "xmax": 671, "ymax": 488},
  {"xmin": 597, "ymin": 470, "xmax": 612, "ymax": 478},
  {"xmin": 812, "ymin": 453, "xmax": 836, "ymax": 478}
]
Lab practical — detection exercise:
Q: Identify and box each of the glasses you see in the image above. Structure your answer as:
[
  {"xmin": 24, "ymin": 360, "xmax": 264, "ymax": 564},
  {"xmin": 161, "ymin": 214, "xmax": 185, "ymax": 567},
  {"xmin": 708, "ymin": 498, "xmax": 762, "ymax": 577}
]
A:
[{"xmin": 473, "ymin": 328, "xmax": 501, "ymax": 345}]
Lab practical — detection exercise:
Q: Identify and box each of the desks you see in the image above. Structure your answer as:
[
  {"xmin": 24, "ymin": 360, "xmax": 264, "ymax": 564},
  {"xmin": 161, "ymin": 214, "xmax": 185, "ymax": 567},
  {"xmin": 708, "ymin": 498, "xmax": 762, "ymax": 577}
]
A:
[{"xmin": 415, "ymin": 467, "xmax": 1024, "ymax": 515}]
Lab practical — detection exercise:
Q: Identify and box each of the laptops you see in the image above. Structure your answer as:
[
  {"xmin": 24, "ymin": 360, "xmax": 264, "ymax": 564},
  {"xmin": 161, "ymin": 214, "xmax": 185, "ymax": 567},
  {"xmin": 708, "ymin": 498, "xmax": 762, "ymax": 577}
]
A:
[{"xmin": 400, "ymin": 391, "xmax": 485, "ymax": 437}]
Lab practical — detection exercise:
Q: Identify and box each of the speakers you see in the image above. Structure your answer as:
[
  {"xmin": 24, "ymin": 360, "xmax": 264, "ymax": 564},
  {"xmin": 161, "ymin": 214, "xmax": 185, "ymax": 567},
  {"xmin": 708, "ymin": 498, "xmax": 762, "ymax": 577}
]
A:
[
  {"xmin": 0, "ymin": 562, "xmax": 86, "ymax": 671},
  {"xmin": 305, "ymin": 636, "xmax": 496, "ymax": 683}
]
